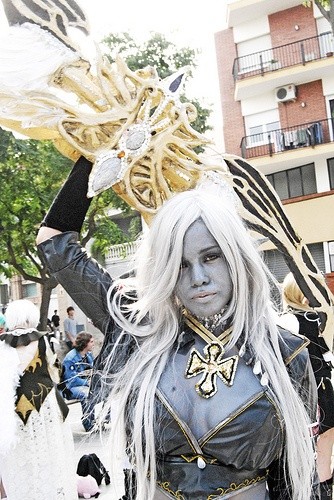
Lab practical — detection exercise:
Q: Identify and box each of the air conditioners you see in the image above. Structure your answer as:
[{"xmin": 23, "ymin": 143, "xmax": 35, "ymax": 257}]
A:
[{"xmin": 275, "ymin": 83, "xmax": 297, "ymax": 103}]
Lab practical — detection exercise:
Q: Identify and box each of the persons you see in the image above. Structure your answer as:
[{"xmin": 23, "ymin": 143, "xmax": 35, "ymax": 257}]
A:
[
  {"xmin": 36, "ymin": 155, "xmax": 334, "ymax": 500},
  {"xmin": 282, "ymin": 272, "xmax": 334, "ymax": 500},
  {"xmin": 0, "ymin": 299, "xmax": 95, "ymax": 500}
]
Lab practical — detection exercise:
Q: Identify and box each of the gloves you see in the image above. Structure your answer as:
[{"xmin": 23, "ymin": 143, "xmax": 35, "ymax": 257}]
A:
[{"xmin": 39, "ymin": 155, "xmax": 95, "ymax": 235}]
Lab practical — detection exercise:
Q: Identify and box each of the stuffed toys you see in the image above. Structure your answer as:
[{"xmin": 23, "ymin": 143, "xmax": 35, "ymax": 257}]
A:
[{"xmin": 76, "ymin": 474, "xmax": 102, "ymax": 499}]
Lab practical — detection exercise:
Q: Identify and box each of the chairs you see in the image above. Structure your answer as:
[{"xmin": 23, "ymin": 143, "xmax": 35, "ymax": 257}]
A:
[{"xmin": 55, "ymin": 382, "xmax": 81, "ymax": 422}]
